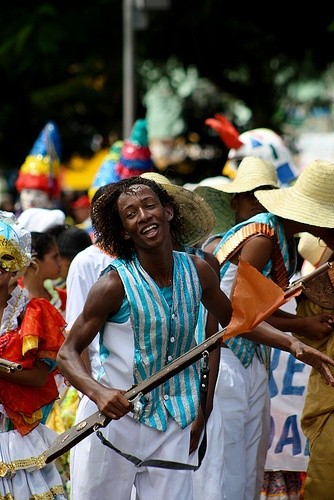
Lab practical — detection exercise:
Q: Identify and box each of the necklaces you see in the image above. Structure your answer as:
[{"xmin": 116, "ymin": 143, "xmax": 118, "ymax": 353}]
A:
[{"xmin": 143, "ymin": 262, "xmax": 173, "ymax": 288}]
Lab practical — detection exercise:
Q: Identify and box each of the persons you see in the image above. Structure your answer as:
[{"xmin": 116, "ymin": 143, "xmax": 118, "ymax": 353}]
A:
[
  {"xmin": 55, "ymin": 176, "xmax": 334, "ymax": 500},
  {"xmin": 0, "ymin": 115, "xmax": 334, "ymax": 500}
]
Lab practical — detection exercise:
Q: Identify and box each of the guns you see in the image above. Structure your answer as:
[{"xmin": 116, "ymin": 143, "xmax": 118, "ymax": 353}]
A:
[{"xmin": 43, "ymin": 259, "xmax": 333, "ymax": 464}]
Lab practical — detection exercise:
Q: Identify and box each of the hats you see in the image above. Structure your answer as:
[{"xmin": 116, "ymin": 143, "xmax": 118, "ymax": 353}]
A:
[
  {"xmin": 206, "ymin": 114, "xmax": 301, "ymax": 188},
  {"xmin": 297, "ymin": 233, "xmax": 334, "ymax": 269},
  {"xmin": 211, "ymin": 156, "xmax": 280, "ymax": 193},
  {"xmin": 139, "ymin": 172, "xmax": 216, "ymax": 247},
  {"xmin": 89, "ymin": 140, "xmax": 125, "ymax": 206},
  {"xmin": 15, "ymin": 122, "xmax": 62, "ymax": 200},
  {"xmin": 115, "ymin": 119, "xmax": 154, "ymax": 179},
  {"xmin": 254, "ymin": 159, "xmax": 334, "ymax": 228},
  {"xmin": 70, "ymin": 195, "xmax": 89, "ymax": 209}
]
